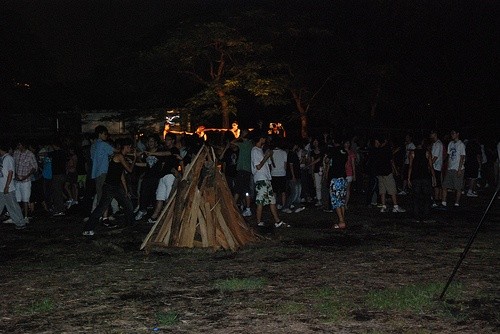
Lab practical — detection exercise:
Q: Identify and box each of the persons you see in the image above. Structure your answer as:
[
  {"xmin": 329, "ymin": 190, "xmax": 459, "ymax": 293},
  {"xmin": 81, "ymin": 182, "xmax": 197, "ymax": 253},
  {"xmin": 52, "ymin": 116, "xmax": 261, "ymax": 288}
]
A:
[
  {"xmin": 0, "ymin": 133, "xmax": 97, "ymax": 229},
  {"xmin": 83, "ymin": 125, "xmax": 206, "ymax": 234},
  {"xmin": 231, "ymin": 120, "xmax": 240, "ymax": 138},
  {"xmin": 353, "ymin": 128, "xmax": 500, "ymax": 212},
  {"xmin": 201, "ymin": 128, "xmax": 356, "ymax": 229}
]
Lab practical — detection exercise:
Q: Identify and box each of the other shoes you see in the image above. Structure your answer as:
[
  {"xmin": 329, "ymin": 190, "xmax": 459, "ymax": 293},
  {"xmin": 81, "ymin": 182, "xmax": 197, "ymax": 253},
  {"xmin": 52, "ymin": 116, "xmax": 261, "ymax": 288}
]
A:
[
  {"xmin": 115, "ymin": 211, "xmax": 124, "ymax": 216},
  {"xmin": 146, "ymin": 216, "xmax": 157, "ymax": 223},
  {"xmin": 368, "ymin": 202, "xmax": 377, "ymax": 207},
  {"xmin": 277, "ymin": 201, "xmax": 334, "ymax": 213},
  {"xmin": 275, "ymin": 221, "xmax": 291, "ymax": 228},
  {"xmin": 82, "ymin": 229, "xmax": 94, "ymax": 236},
  {"xmin": 454, "ymin": 204, "xmax": 459, "ymax": 208},
  {"xmin": 392, "ymin": 207, "xmax": 406, "ymax": 213},
  {"xmin": 435, "ymin": 204, "xmax": 448, "ymax": 210},
  {"xmin": 485, "ymin": 184, "xmax": 489, "ymax": 190},
  {"xmin": 135, "ymin": 210, "xmax": 147, "ymax": 221},
  {"xmin": 467, "ymin": 193, "xmax": 478, "ymax": 196},
  {"xmin": 380, "ymin": 207, "xmax": 390, "ymax": 213},
  {"xmin": 256, "ymin": 223, "xmax": 265, "ymax": 227},
  {"xmin": 398, "ymin": 191, "xmax": 407, "ymax": 195},
  {"xmin": 2, "ymin": 198, "xmax": 84, "ymax": 231},
  {"xmin": 102, "ymin": 218, "xmax": 109, "ymax": 226},
  {"xmin": 334, "ymin": 223, "xmax": 346, "ymax": 229},
  {"xmin": 241, "ymin": 210, "xmax": 252, "ymax": 216}
]
